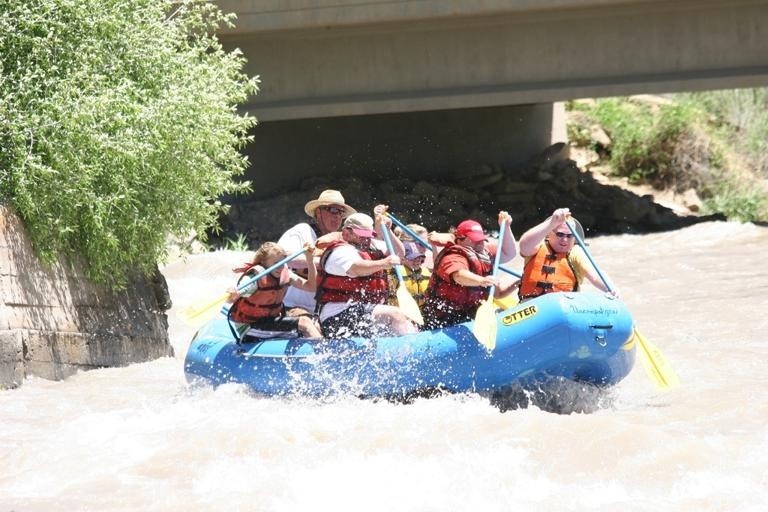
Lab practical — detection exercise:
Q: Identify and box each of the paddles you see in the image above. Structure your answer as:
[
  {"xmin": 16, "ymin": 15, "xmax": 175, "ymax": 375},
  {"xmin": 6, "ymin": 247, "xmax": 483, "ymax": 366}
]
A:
[
  {"xmin": 564, "ymin": 217, "xmax": 676, "ymax": 391},
  {"xmin": 388, "ymin": 214, "xmax": 518, "ymax": 310},
  {"xmin": 472, "ymin": 217, "xmax": 507, "ymax": 351},
  {"xmin": 381, "ymin": 216, "xmax": 423, "ymax": 323},
  {"xmin": 188, "ymin": 245, "xmax": 307, "ymax": 322}
]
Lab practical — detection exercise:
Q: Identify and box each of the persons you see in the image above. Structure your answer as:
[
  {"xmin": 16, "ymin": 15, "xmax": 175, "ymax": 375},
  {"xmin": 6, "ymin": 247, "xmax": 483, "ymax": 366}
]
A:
[
  {"xmin": 420, "ymin": 209, "xmax": 518, "ymax": 332},
  {"xmin": 273, "ymin": 185, "xmax": 347, "ymax": 313},
  {"xmin": 227, "ymin": 240, "xmax": 323, "ymax": 341},
  {"xmin": 518, "ymin": 207, "xmax": 620, "ymax": 307},
  {"xmin": 315, "ymin": 211, "xmax": 420, "ymax": 338},
  {"xmin": 392, "ymin": 214, "xmax": 439, "ymax": 277}
]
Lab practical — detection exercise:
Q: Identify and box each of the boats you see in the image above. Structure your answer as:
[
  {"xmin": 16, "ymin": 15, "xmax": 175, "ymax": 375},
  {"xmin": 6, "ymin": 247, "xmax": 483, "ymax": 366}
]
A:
[{"xmin": 184, "ymin": 291, "xmax": 636, "ymax": 399}]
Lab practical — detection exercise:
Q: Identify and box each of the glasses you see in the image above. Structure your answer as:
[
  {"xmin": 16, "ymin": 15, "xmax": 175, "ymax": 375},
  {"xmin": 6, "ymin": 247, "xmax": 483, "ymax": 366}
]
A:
[
  {"xmin": 552, "ymin": 230, "xmax": 574, "ymax": 238},
  {"xmin": 320, "ymin": 206, "xmax": 345, "ymax": 215}
]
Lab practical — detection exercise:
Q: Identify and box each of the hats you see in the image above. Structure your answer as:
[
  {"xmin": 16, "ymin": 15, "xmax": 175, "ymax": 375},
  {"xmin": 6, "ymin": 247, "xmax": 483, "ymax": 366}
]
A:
[
  {"xmin": 304, "ymin": 190, "xmax": 358, "ymax": 219},
  {"xmin": 343, "ymin": 213, "xmax": 375, "ymax": 238},
  {"xmin": 457, "ymin": 220, "xmax": 489, "ymax": 242},
  {"xmin": 402, "ymin": 241, "xmax": 425, "ymax": 260}
]
